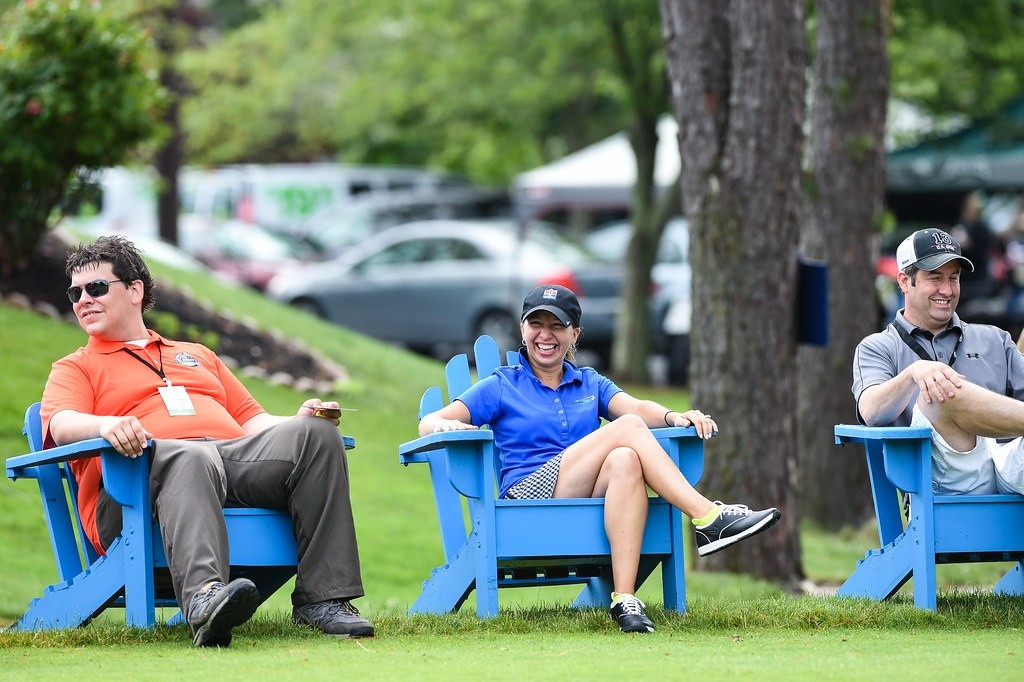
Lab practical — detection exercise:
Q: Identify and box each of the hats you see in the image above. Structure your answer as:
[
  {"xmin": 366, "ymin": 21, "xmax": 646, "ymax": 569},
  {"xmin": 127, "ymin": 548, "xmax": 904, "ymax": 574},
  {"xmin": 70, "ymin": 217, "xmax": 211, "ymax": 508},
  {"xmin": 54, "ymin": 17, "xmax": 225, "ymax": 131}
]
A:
[
  {"xmin": 896, "ymin": 228, "xmax": 975, "ymax": 273},
  {"xmin": 520, "ymin": 284, "xmax": 582, "ymax": 328}
]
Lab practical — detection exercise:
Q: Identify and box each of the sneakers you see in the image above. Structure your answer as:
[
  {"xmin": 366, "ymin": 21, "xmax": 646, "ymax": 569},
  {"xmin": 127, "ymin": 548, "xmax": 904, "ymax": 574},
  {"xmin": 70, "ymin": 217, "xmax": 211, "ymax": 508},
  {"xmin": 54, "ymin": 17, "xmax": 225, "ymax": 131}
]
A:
[
  {"xmin": 609, "ymin": 592, "xmax": 656, "ymax": 633},
  {"xmin": 188, "ymin": 577, "xmax": 260, "ymax": 649},
  {"xmin": 292, "ymin": 598, "xmax": 375, "ymax": 640},
  {"xmin": 692, "ymin": 500, "xmax": 782, "ymax": 555}
]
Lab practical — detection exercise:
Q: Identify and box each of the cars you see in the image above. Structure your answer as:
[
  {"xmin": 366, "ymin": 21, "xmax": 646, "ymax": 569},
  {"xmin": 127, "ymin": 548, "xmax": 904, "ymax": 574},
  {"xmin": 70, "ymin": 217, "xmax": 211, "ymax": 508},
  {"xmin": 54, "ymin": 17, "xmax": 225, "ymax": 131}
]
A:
[
  {"xmin": 580, "ymin": 212, "xmax": 692, "ymax": 390},
  {"xmin": 264, "ymin": 217, "xmax": 675, "ymax": 371}
]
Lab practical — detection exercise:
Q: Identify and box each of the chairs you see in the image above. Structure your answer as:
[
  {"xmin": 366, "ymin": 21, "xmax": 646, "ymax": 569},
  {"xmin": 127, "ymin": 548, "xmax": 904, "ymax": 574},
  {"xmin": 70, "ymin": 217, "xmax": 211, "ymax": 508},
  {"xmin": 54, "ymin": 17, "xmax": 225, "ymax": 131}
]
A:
[
  {"xmin": 5, "ymin": 402, "xmax": 356, "ymax": 617},
  {"xmin": 396, "ymin": 334, "xmax": 716, "ymax": 617},
  {"xmin": 833, "ymin": 422, "xmax": 1024, "ymax": 610}
]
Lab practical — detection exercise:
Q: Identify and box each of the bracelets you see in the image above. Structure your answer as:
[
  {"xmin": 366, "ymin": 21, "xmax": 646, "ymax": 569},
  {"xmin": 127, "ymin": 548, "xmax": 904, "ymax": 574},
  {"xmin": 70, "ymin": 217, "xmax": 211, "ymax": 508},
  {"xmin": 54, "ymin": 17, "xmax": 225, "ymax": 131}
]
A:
[{"xmin": 664, "ymin": 411, "xmax": 675, "ymax": 427}]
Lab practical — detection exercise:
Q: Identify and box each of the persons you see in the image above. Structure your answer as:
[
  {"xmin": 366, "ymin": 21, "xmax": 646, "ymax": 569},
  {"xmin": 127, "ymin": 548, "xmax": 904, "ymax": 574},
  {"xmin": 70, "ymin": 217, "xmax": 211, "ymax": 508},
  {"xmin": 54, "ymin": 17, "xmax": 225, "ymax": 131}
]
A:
[
  {"xmin": 853, "ymin": 228, "xmax": 1024, "ymax": 498},
  {"xmin": 418, "ymin": 285, "xmax": 780, "ymax": 632},
  {"xmin": 41, "ymin": 236, "xmax": 376, "ymax": 645}
]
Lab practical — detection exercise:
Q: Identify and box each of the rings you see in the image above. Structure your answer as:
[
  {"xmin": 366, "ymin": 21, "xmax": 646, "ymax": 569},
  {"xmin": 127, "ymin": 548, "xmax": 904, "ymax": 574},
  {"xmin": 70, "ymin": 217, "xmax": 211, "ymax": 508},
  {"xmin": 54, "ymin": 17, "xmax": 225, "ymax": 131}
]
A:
[{"xmin": 705, "ymin": 415, "xmax": 711, "ymax": 418}]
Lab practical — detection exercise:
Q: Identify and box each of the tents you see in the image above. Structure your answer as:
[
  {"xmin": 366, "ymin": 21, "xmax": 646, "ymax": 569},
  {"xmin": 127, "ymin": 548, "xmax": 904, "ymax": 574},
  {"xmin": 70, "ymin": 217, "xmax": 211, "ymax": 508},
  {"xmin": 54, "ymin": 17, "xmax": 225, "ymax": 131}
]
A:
[{"xmin": 510, "ymin": 79, "xmax": 1024, "ymax": 380}]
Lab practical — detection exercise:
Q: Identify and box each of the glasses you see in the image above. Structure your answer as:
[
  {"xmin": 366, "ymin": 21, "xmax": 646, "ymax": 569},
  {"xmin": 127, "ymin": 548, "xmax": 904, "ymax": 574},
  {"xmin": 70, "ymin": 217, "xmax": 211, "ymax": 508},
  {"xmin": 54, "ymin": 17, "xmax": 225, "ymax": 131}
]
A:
[{"xmin": 66, "ymin": 279, "xmax": 122, "ymax": 303}]
